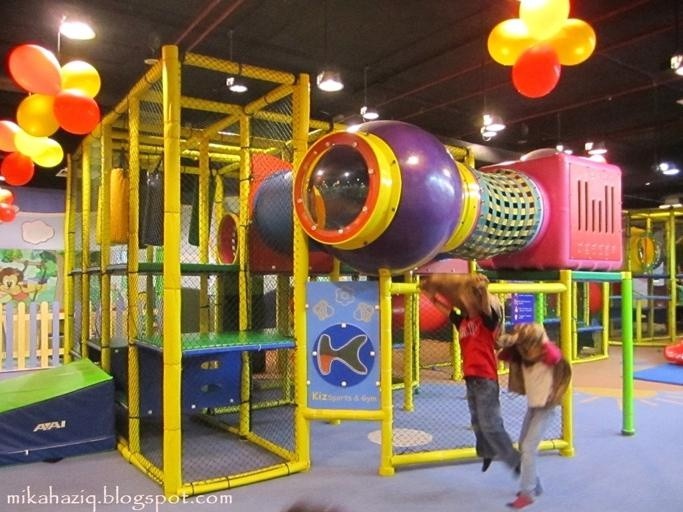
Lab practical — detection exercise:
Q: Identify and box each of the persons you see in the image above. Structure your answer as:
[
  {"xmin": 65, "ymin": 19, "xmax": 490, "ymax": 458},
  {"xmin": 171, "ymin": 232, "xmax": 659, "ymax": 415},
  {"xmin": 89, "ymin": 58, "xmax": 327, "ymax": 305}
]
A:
[
  {"xmin": 416, "ymin": 270, "xmax": 542, "ymax": 497},
  {"xmin": 498, "ymin": 319, "xmax": 571, "ymax": 509}
]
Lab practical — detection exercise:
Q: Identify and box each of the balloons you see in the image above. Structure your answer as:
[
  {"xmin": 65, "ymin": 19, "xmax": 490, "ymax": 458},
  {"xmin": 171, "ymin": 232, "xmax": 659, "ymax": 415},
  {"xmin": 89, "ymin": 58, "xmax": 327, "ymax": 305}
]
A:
[
  {"xmin": 487, "ymin": 17, "xmax": 540, "ymax": 66},
  {"xmin": 549, "ymin": 17, "xmax": 599, "ymax": 66},
  {"xmin": 519, "ymin": 0, "xmax": 571, "ymax": 42},
  {"xmin": 1, "ymin": 43, "xmax": 103, "ymax": 225},
  {"xmin": 511, "ymin": 41, "xmax": 562, "ymax": 99}
]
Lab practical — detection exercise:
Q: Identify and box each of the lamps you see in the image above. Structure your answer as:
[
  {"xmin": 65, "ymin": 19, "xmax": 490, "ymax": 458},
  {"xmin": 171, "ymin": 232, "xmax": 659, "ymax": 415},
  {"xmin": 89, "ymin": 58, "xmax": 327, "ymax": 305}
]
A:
[
  {"xmin": 318, "ymin": 0, "xmax": 345, "ymax": 93},
  {"xmin": 480, "ymin": 89, "xmax": 506, "ymax": 140},
  {"xmin": 583, "ymin": 133, "xmax": 607, "ymax": 159},
  {"xmin": 361, "ymin": 64, "xmax": 379, "ymax": 121},
  {"xmin": 227, "ymin": 31, "xmax": 246, "ymax": 93}
]
